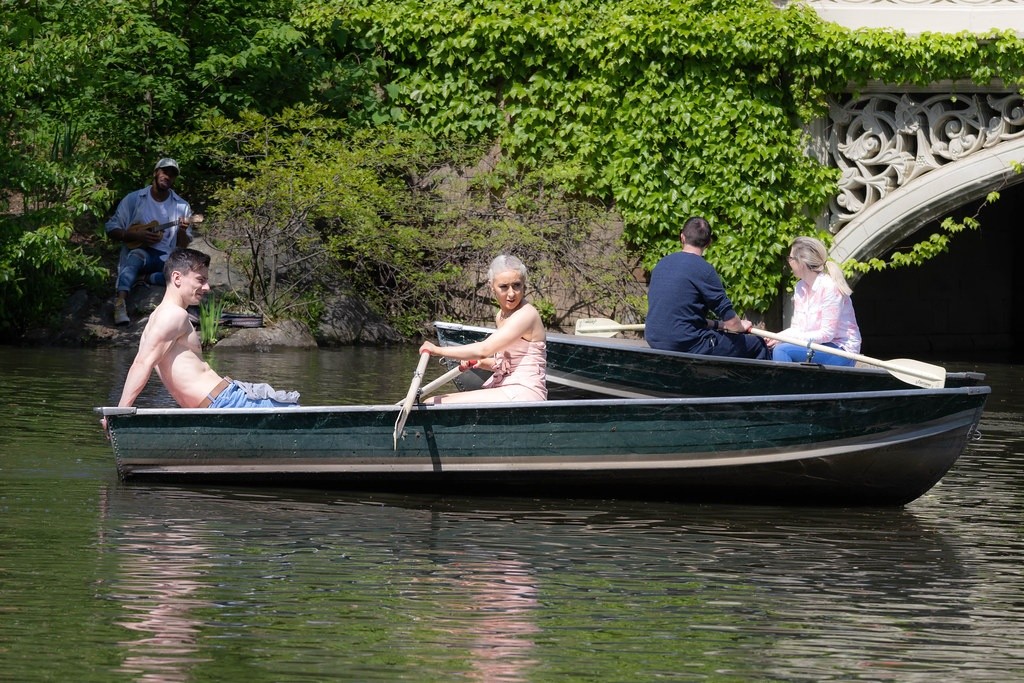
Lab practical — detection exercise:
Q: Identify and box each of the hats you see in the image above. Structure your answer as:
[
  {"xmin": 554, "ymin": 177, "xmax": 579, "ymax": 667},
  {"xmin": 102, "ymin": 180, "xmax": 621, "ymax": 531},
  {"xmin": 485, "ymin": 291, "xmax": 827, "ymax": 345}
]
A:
[{"xmin": 154, "ymin": 158, "xmax": 180, "ymax": 176}]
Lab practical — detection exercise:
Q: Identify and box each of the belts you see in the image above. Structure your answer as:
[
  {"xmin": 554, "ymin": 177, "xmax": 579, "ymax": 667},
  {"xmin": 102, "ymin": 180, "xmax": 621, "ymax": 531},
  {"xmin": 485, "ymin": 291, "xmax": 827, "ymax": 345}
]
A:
[{"xmin": 199, "ymin": 375, "xmax": 233, "ymax": 408}]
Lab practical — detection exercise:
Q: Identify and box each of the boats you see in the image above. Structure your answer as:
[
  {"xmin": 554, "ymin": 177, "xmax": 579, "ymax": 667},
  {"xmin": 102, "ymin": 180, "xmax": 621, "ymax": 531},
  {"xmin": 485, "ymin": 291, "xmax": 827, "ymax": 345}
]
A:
[
  {"xmin": 95, "ymin": 385, "xmax": 993, "ymax": 511},
  {"xmin": 435, "ymin": 322, "xmax": 988, "ymax": 403}
]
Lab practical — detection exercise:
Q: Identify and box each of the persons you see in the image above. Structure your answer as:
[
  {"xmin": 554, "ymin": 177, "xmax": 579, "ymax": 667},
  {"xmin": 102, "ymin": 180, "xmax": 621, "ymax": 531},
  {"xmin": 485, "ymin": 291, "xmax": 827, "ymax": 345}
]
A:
[
  {"xmin": 765, "ymin": 235, "xmax": 863, "ymax": 366},
  {"xmin": 101, "ymin": 247, "xmax": 302, "ymax": 439},
  {"xmin": 644, "ymin": 216, "xmax": 770, "ymax": 360},
  {"xmin": 105, "ymin": 157, "xmax": 194, "ymax": 323},
  {"xmin": 419, "ymin": 254, "xmax": 549, "ymax": 405}
]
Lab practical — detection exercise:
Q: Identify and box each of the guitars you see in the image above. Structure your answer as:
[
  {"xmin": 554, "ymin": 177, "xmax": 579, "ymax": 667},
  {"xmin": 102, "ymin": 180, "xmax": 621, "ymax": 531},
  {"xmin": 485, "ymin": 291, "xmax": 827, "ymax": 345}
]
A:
[{"xmin": 126, "ymin": 213, "xmax": 204, "ymax": 250}]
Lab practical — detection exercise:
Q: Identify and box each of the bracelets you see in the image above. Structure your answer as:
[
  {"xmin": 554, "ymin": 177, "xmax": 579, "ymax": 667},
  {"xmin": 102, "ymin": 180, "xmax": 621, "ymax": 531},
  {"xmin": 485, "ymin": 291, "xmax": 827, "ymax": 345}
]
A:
[{"xmin": 713, "ymin": 319, "xmax": 719, "ymax": 329}]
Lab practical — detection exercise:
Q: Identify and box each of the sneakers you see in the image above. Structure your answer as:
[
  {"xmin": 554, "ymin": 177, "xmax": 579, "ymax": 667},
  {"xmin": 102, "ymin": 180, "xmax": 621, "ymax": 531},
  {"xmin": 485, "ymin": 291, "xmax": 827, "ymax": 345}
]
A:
[{"xmin": 114, "ymin": 297, "xmax": 130, "ymax": 326}]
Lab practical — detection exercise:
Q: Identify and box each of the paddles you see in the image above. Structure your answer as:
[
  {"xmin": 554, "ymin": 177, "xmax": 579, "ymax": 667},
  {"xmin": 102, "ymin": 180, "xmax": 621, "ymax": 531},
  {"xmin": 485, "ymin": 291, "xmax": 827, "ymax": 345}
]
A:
[
  {"xmin": 394, "ymin": 350, "xmax": 430, "ymax": 450},
  {"xmin": 393, "ymin": 360, "xmax": 477, "ymax": 407},
  {"xmin": 574, "ymin": 318, "xmax": 646, "ymax": 339},
  {"xmin": 748, "ymin": 327, "xmax": 946, "ymax": 390}
]
